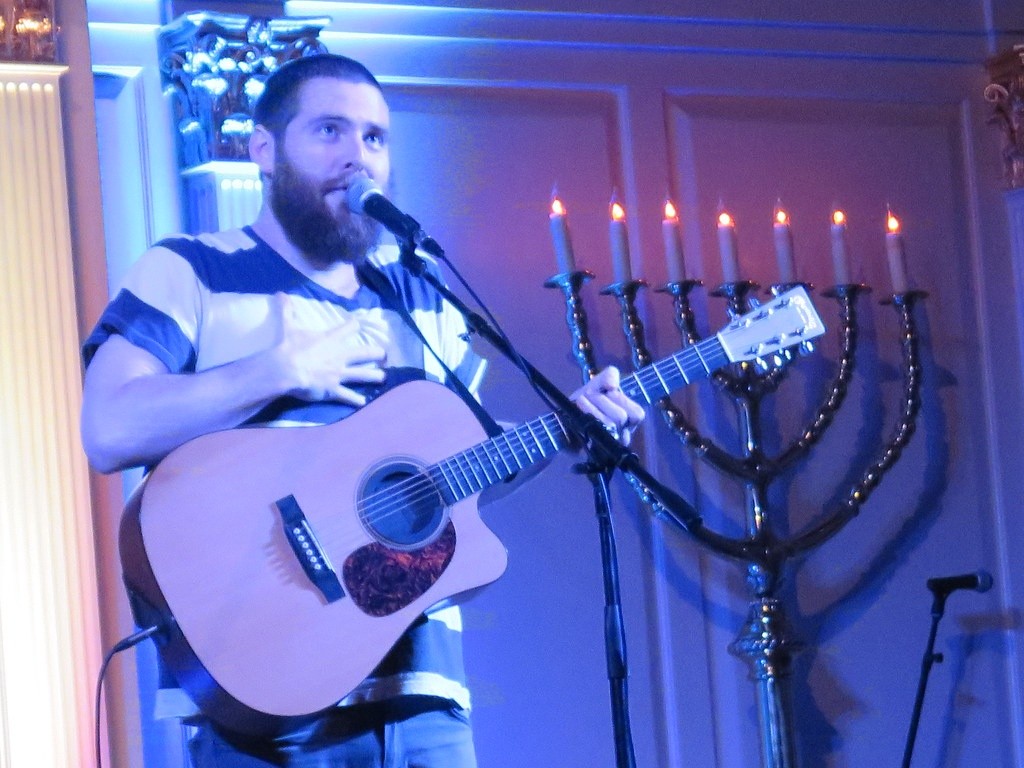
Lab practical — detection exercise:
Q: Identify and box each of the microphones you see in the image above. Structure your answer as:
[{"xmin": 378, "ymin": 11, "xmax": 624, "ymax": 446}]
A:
[
  {"xmin": 346, "ymin": 178, "xmax": 444, "ymax": 258},
  {"xmin": 927, "ymin": 570, "xmax": 993, "ymax": 592}
]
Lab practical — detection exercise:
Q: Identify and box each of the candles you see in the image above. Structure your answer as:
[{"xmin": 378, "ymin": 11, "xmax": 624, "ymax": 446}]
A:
[
  {"xmin": 661, "ymin": 199, "xmax": 688, "ymax": 283},
  {"xmin": 549, "ymin": 196, "xmax": 577, "ymax": 274},
  {"xmin": 830, "ymin": 209, "xmax": 853, "ymax": 286},
  {"xmin": 715, "ymin": 214, "xmax": 744, "ymax": 283},
  {"xmin": 772, "ymin": 211, "xmax": 797, "ymax": 282},
  {"xmin": 609, "ymin": 203, "xmax": 633, "ymax": 283},
  {"xmin": 883, "ymin": 209, "xmax": 908, "ymax": 293}
]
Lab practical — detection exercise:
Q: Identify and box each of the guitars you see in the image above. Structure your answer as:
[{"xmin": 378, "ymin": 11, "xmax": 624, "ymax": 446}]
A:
[{"xmin": 117, "ymin": 279, "xmax": 827, "ymax": 742}]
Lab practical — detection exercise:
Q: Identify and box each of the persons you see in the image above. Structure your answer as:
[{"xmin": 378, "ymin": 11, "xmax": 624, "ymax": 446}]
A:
[{"xmin": 79, "ymin": 54, "xmax": 647, "ymax": 768}]
